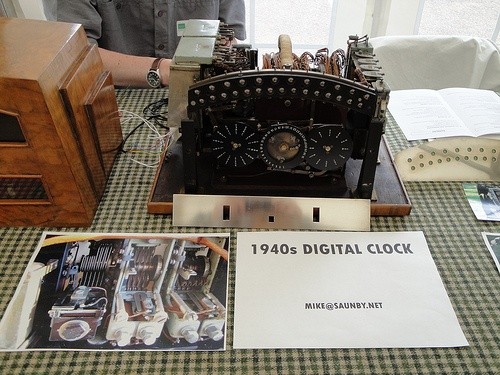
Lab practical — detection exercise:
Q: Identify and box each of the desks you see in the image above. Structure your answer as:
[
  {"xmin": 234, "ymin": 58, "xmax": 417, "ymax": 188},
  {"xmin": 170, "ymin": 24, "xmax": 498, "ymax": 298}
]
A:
[{"xmin": 0, "ymin": 83, "xmax": 500, "ymax": 375}]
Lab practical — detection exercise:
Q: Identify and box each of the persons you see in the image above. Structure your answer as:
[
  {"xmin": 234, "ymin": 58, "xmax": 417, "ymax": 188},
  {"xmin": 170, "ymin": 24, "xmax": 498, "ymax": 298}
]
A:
[{"xmin": 56, "ymin": 0, "xmax": 246, "ymax": 90}]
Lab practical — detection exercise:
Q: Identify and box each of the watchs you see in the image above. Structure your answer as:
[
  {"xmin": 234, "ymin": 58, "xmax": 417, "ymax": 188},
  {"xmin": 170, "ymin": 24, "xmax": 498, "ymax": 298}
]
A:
[{"xmin": 146, "ymin": 58, "xmax": 165, "ymax": 89}]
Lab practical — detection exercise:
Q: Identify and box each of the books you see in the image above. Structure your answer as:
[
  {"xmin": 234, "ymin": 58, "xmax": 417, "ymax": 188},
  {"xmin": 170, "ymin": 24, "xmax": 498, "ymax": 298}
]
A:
[{"xmin": 386, "ymin": 88, "xmax": 500, "ymax": 141}]
